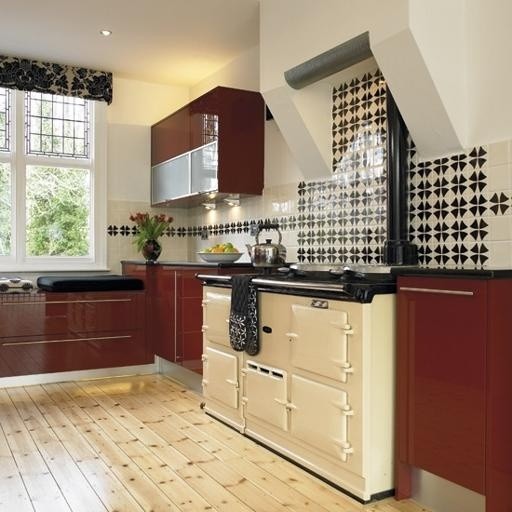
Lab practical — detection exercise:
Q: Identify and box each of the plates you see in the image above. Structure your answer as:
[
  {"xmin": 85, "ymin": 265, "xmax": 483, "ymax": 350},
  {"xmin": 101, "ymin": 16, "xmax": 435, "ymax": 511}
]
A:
[{"xmin": 195, "ymin": 250, "xmax": 244, "ymax": 264}]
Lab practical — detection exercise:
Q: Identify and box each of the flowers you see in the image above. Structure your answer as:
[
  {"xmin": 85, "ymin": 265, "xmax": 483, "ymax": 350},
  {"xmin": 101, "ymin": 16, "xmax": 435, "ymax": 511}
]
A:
[{"xmin": 129, "ymin": 212, "xmax": 174, "ymax": 253}]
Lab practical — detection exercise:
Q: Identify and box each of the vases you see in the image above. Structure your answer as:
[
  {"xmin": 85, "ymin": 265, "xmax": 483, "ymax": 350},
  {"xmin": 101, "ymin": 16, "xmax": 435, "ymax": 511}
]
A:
[{"xmin": 142, "ymin": 240, "xmax": 161, "ymax": 261}]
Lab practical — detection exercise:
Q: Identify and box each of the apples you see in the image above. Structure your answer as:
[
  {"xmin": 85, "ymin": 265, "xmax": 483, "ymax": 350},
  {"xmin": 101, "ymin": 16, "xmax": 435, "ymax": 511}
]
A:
[{"xmin": 199, "ymin": 242, "xmax": 238, "ymax": 253}]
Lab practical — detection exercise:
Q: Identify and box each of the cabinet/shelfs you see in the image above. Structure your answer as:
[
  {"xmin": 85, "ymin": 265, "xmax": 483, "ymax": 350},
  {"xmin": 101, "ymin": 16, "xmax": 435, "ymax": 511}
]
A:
[
  {"xmin": 390, "ymin": 267, "xmax": 510, "ymax": 512},
  {"xmin": 119, "ymin": 264, "xmax": 200, "ymax": 387},
  {"xmin": 151, "ymin": 86, "xmax": 264, "ymax": 209},
  {"xmin": 194, "ymin": 273, "xmax": 390, "ymax": 500},
  {"xmin": 4, "ymin": 289, "xmax": 149, "ymax": 388}
]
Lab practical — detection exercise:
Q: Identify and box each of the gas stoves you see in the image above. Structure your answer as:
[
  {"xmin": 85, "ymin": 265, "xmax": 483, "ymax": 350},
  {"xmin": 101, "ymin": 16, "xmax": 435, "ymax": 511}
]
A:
[{"xmin": 241, "ymin": 269, "xmax": 390, "ymax": 288}]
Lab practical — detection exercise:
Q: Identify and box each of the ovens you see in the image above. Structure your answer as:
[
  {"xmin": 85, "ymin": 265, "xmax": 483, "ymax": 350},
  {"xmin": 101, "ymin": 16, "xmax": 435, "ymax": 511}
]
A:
[{"xmin": 202, "ymin": 285, "xmax": 355, "ymax": 463}]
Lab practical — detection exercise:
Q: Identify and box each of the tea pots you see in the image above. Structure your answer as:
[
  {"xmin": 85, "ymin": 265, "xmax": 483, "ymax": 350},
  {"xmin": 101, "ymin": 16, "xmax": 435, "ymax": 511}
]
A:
[{"xmin": 244, "ymin": 222, "xmax": 288, "ymax": 268}]
